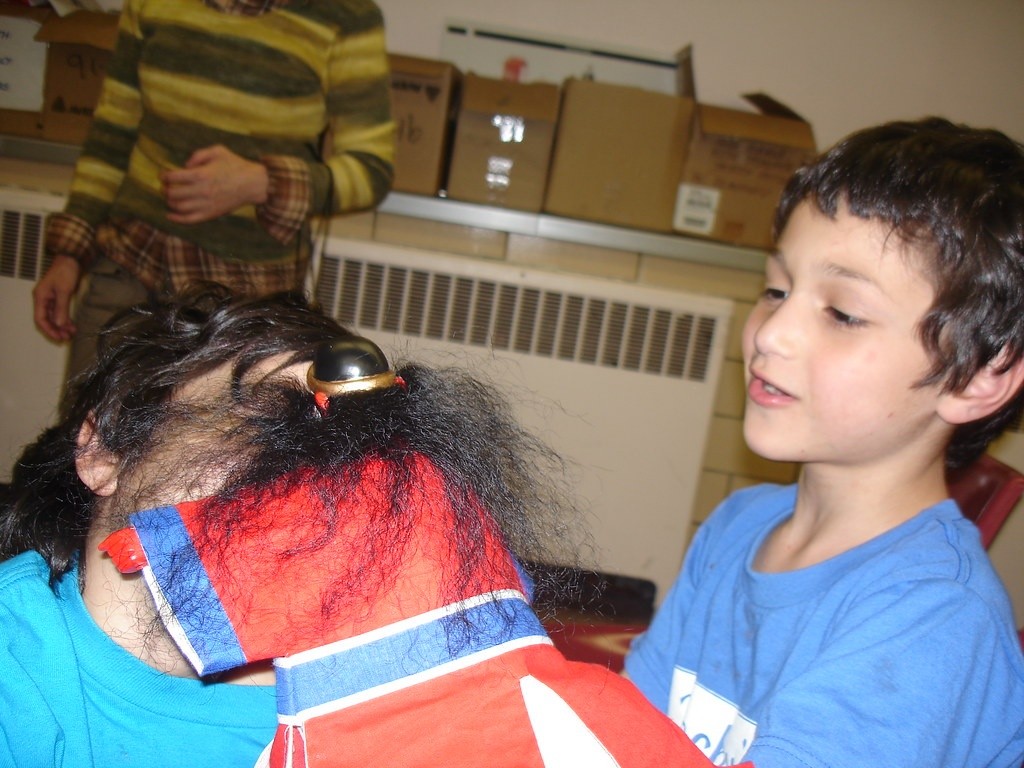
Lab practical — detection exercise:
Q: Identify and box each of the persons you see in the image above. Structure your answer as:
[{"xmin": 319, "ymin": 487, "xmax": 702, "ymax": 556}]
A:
[
  {"xmin": 34, "ymin": 1, "xmax": 399, "ymax": 416},
  {"xmin": 624, "ymin": 116, "xmax": 1024, "ymax": 768},
  {"xmin": 4, "ymin": 279, "xmax": 353, "ymax": 768},
  {"xmin": 110, "ymin": 336, "xmax": 713, "ymax": 768}
]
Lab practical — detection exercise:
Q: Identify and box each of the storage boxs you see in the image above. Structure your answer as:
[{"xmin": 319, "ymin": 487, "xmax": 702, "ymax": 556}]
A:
[
  {"xmin": 447, "ymin": 73, "xmax": 562, "ymax": 212},
  {"xmin": 547, "ymin": 76, "xmax": 696, "ymax": 233},
  {"xmin": 320, "ymin": 51, "xmax": 463, "ymax": 195},
  {"xmin": 674, "ymin": 103, "xmax": 818, "ymax": 250},
  {"xmin": 37, "ymin": 10, "xmax": 120, "ymax": 108}
]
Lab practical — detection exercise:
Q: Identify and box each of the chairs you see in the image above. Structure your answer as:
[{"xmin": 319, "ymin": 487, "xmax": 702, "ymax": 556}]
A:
[{"xmin": 944, "ymin": 451, "xmax": 1024, "ymax": 551}]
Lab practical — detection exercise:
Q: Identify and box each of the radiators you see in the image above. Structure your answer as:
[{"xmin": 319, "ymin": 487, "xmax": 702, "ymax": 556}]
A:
[{"xmin": 0, "ymin": 186, "xmax": 734, "ymax": 615}]
